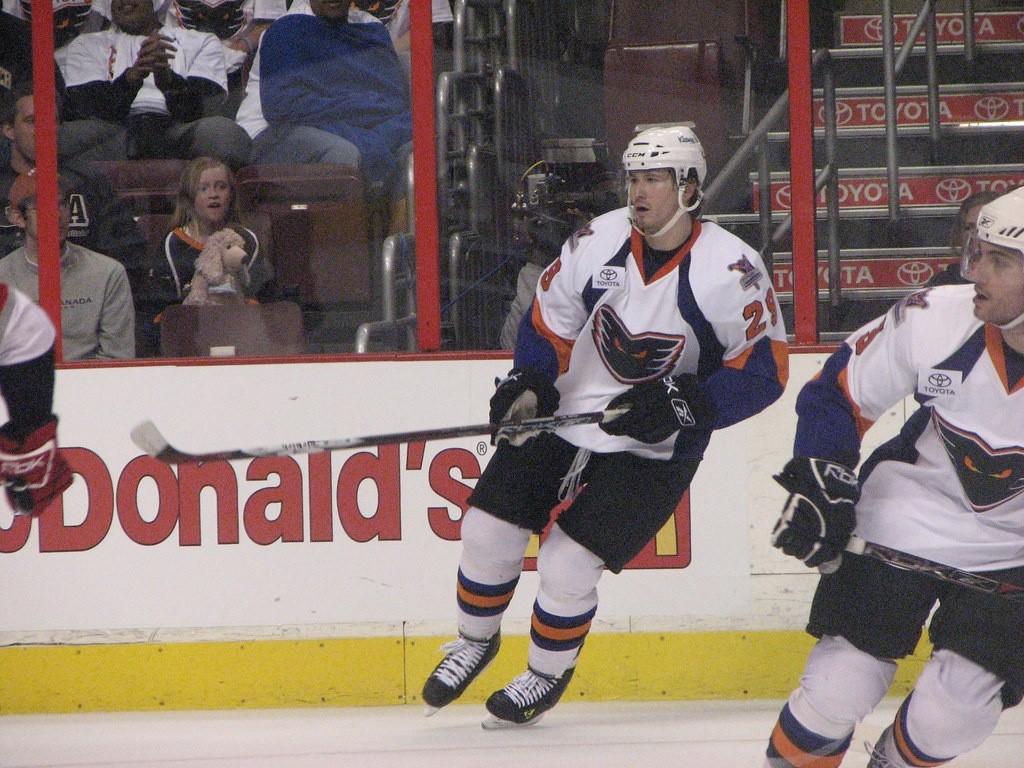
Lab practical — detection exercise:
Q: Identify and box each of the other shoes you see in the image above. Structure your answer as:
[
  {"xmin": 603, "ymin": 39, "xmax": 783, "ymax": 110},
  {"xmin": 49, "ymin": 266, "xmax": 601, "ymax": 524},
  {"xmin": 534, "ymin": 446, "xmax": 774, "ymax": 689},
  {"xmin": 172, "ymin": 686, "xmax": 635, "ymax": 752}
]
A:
[{"xmin": 864, "ymin": 724, "xmax": 906, "ymax": 768}]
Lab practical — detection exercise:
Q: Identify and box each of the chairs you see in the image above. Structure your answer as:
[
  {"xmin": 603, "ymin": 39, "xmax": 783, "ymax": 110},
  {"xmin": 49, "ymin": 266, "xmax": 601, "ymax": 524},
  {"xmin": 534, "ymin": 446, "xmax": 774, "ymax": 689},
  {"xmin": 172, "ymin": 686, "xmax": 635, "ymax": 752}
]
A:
[
  {"xmin": 231, "ymin": 161, "xmax": 393, "ymax": 303},
  {"xmin": 158, "ymin": 301, "xmax": 308, "ymax": 356},
  {"xmin": 88, "ymin": 159, "xmax": 189, "ymax": 267}
]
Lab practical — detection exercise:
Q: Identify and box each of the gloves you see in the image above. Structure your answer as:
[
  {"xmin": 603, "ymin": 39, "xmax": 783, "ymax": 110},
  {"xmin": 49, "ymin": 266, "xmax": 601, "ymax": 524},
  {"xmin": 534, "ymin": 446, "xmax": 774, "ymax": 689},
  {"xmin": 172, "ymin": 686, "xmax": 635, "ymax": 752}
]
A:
[
  {"xmin": 0, "ymin": 415, "xmax": 73, "ymax": 516},
  {"xmin": 598, "ymin": 372, "xmax": 715, "ymax": 445},
  {"xmin": 489, "ymin": 367, "xmax": 560, "ymax": 451},
  {"xmin": 770, "ymin": 455, "xmax": 861, "ymax": 568}
]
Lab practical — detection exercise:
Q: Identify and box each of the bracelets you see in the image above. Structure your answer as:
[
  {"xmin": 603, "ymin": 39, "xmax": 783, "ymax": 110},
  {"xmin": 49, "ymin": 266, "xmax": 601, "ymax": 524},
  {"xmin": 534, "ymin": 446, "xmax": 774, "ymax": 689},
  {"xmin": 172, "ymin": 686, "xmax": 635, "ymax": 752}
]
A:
[{"xmin": 240, "ymin": 37, "xmax": 254, "ymax": 54}]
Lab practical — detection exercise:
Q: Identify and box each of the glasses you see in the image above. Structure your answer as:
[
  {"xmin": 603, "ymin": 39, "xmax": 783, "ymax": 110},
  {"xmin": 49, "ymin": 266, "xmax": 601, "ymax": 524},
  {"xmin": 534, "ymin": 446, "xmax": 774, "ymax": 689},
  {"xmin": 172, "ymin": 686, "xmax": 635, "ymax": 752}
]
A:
[{"xmin": 20, "ymin": 200, "xmax": 70, "ymax": 212}]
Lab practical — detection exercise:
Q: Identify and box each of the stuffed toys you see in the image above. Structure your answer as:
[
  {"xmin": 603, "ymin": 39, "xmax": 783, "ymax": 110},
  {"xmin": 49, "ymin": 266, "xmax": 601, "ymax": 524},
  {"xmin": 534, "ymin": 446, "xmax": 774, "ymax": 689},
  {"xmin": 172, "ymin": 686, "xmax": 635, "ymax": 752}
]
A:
[{"xmin": 184, "ymin": 229, "xmax": 250, "ymax": 305}]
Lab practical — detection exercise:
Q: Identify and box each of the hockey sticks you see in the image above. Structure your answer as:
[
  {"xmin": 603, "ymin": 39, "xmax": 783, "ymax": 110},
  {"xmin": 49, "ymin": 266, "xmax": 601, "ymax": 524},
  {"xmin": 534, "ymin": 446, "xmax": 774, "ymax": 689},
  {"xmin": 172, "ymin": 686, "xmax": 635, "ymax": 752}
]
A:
[
  {"xmin": 843, "ymin": 536, "xmax": 1024, "ymax": 604},
  {"xmin": 130, "ymin": 403, "xmax": 637, "ymax": 467}
]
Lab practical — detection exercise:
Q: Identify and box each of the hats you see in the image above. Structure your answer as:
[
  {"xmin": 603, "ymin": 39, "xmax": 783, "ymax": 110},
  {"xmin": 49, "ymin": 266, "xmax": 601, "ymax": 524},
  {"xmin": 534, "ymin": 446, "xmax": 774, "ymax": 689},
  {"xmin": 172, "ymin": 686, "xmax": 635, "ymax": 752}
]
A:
[{"xmin": 10, "ymin": 168, "xmax": 72, "ymax": 210}]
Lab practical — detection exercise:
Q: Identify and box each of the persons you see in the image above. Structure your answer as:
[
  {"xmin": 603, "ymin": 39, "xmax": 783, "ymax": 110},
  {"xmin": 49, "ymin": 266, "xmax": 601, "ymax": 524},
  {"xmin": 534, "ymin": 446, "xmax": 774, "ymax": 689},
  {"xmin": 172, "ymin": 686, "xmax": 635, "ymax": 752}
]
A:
[
  {"xmin": 501, "ymin": 207, "xmax": 594, "ymax": 351},
  {"xmin": 0, "ymin": 168, "xmax": 135, "ymax": 359},
  {"xmin": 155, "ymin": 158, "xmax": 276, "ymax": 313},
  {"xmin": 0, "ymin": 282, "xmax": 76, "ymax": 512},
  {"xmin": 0, "ymin": 0, "xmax": 454, "ymax": 274},
  {"xmin": 924, "ymin": 190, "xmax": 997, "ymax": 289},
  {"xmin": 421, "ymin": 126, "xmax": 790, "ymax": 731},
  {"xmin": 762, "ymin": 183, "xmax": 1023, "ymax": 768}
]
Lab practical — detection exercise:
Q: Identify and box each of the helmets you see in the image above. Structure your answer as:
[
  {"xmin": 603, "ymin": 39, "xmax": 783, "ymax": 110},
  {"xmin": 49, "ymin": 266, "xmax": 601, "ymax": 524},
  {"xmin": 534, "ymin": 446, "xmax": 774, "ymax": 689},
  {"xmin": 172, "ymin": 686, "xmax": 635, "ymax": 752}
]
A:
[
  {"xmin": 976, "ymin": 186, "xmax": 1024, "ymax": 254},
  {"xmin": 622, "ymin": 126, "xmax": 708, "ymax": 186}
]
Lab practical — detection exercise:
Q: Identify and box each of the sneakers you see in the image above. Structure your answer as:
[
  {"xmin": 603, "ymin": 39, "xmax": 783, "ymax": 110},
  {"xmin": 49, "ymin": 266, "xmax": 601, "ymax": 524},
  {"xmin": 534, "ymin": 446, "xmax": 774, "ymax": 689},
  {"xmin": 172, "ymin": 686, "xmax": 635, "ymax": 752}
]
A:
[
  {"xmin": 422, "ymin": 624, "xmax": 502, "ymax": 717},
  {"xmin": 481, "ymin": 666, "xmax": 576, "ymax": 730}
]
